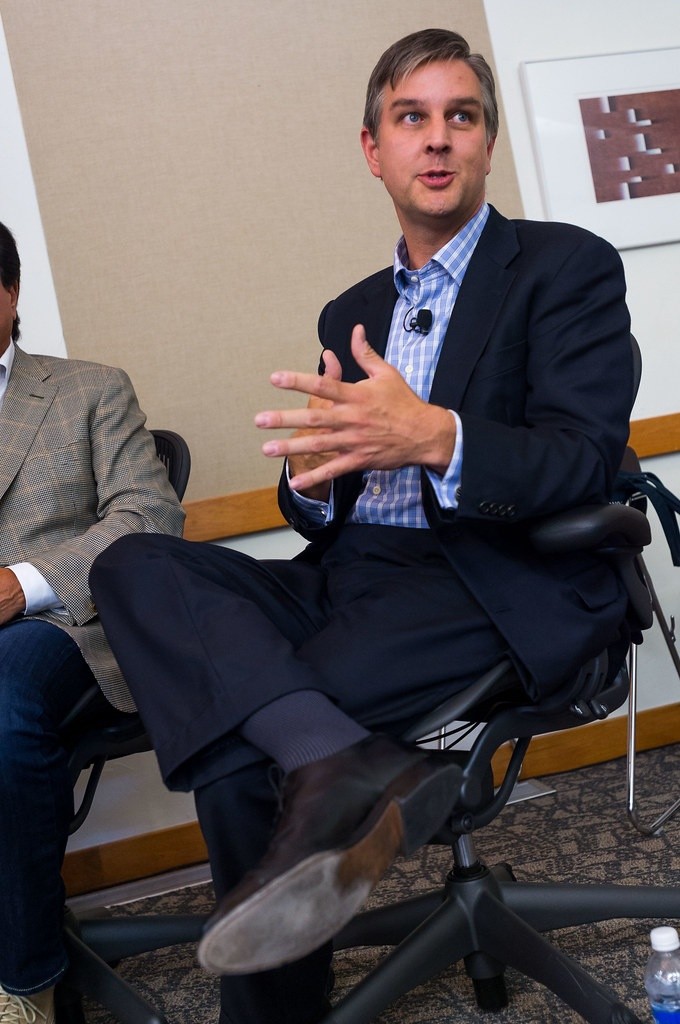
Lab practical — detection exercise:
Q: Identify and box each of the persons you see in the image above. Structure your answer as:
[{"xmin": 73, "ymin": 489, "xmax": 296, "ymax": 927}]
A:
[
  {"xmin": 88, "ymin": 30, "xmax": 643, "ymax": 1023},
  {"xmin": 0, "ymin": 220, "xmax": 184, "ymax": 1022}
]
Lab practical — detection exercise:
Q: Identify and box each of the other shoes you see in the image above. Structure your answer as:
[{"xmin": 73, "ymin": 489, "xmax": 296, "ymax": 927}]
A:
[{"xmin": 0, "ymin": 987, "xmax": 54, "ymax": 1024}]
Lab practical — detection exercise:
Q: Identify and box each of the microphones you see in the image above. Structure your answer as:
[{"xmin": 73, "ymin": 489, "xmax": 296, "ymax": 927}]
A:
[{"xmin": 409, "ymin": 309, "xmax": 432, "ymax": 335}]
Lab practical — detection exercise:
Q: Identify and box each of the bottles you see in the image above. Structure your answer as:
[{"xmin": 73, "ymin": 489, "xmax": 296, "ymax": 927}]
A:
[{"xmin": 643, "ymin": 926, "xmax": 680, "ymax": 1024}]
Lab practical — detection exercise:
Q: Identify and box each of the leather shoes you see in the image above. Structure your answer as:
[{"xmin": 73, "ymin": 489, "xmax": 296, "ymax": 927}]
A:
[{"xmin": 193, "ymin": 734, "xmax": 465, "ymax": 976}]
